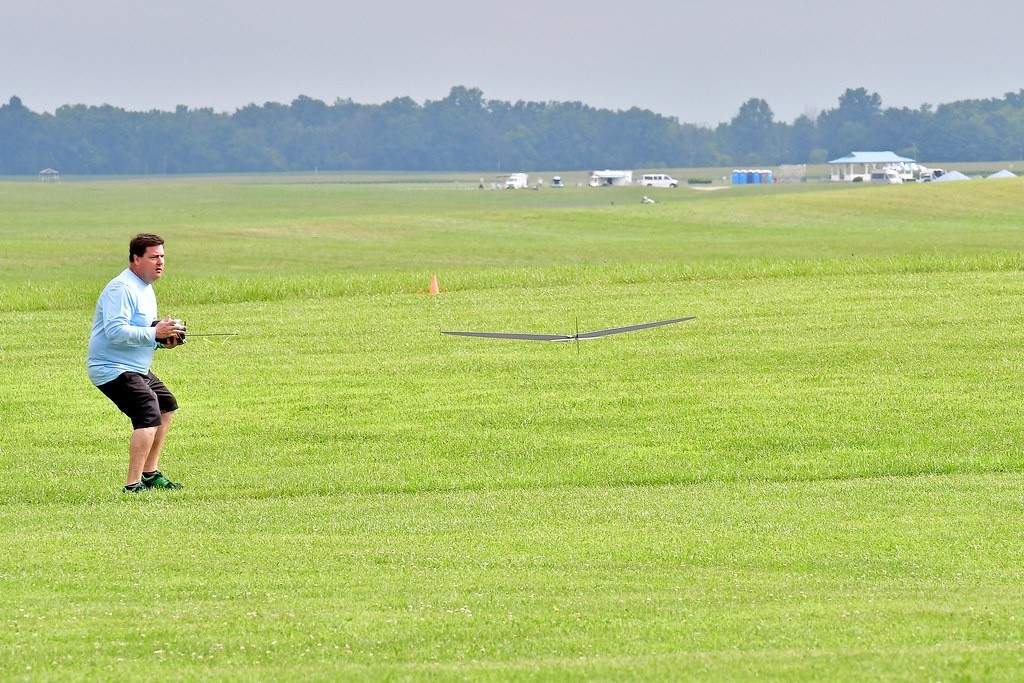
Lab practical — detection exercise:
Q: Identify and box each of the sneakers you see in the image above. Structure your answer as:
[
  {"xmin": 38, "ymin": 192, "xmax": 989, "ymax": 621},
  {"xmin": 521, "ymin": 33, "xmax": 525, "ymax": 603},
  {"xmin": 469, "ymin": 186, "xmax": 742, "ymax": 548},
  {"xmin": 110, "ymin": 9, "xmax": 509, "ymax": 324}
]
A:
[
  {"xmin": 122, "ymin": 481, "xmax": 151, "ymax": 493},
  {"xmin": 141, "ymin": 472, "xmax": 183, "ymax": 489}
]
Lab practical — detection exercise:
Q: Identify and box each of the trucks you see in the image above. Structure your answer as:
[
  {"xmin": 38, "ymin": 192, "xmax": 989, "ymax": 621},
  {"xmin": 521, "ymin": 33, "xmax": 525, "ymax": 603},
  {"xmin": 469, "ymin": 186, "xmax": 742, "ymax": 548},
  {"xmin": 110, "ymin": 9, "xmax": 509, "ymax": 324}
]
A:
[{"xmin": 505, "ymin": 173, "xmax": 527, "ymax": 190}]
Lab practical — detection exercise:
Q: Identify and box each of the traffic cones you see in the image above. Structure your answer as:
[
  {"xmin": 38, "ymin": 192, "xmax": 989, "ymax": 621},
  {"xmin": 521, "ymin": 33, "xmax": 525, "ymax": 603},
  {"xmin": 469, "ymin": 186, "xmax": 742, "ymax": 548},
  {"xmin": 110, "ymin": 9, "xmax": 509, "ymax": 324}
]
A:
[{"xmin": 429, "ymin": 275, "xmax": 439, "ymax": 295}]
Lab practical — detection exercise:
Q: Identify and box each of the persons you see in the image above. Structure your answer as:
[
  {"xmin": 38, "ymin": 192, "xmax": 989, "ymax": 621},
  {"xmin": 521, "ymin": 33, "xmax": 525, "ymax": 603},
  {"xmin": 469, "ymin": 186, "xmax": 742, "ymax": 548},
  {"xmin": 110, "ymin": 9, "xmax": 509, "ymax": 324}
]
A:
[
  {"xmin": 479, "ymin": 176, "xmax": 483, "ymax": 190},
  {"xmin": 87, "ymin": 233, "xmax": 186, "ymax": 493},
  {"xmin": 539, "ymin": 177, "xmax": 543, "ymax": 188}
]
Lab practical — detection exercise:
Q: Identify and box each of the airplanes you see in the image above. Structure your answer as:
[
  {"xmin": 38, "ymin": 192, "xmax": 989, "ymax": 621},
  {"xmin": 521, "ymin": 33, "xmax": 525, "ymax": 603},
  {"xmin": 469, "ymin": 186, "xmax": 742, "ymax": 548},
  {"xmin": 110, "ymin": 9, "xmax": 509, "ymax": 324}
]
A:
[{"xmin": 440, "ymin": 316, "xmax": 697, "ymax": 356}]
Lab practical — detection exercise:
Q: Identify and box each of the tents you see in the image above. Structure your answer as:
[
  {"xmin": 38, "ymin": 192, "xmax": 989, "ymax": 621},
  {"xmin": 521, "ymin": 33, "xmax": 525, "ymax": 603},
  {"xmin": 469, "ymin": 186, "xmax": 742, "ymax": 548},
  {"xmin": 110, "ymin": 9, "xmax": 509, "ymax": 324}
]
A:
[
  {"xmin": 931, "ymin": 170, "xmax": 970, "ymax": 182},
  {"xmin": 985, "ymin": 170, "xmax": 1016, "ymax": 180}
]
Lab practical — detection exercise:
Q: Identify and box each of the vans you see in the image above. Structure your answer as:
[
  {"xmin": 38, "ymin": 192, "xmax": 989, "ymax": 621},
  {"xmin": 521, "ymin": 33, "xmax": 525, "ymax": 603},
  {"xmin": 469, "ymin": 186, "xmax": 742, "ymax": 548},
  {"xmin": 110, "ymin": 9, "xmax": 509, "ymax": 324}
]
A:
[
  {"xmin": 920, "ymin": 168, "xmax": 943, "ymax": 183},
  {"xmin": 641, "ymin": 174, "xmax": 679, "ymax": 188},
  {"xmin": 871, "ymin": 169, "xmax": 902, "ymax": 184}
]
